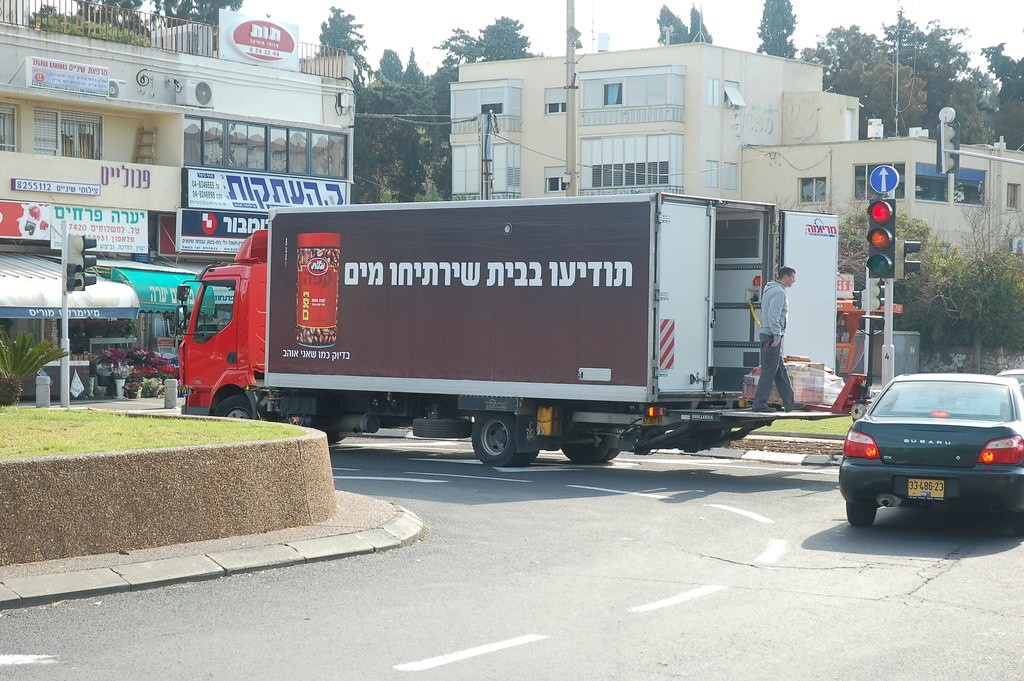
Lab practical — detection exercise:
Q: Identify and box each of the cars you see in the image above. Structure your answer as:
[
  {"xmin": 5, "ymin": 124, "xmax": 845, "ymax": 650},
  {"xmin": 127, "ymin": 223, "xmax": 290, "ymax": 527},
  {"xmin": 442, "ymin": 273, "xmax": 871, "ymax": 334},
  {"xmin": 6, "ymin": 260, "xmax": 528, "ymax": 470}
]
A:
[{"xmin": 838, "ymin": 369, "xmax": 1024, "ymax": 536}]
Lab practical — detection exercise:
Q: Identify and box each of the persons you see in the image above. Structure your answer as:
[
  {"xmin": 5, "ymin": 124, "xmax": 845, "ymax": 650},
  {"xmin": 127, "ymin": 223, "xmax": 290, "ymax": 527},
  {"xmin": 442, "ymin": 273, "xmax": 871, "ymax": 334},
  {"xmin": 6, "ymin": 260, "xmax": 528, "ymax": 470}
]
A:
[{"xmin": 751, "ymin": 267, "xmax": 807, "ymax": 413}]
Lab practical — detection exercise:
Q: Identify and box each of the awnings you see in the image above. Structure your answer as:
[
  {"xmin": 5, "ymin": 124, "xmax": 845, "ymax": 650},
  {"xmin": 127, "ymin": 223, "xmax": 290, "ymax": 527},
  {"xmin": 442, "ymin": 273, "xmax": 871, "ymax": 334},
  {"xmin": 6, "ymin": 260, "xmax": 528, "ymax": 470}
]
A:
[{"xmin": 0, "ymin": 252, "xmax": 216, "ymax": 320}]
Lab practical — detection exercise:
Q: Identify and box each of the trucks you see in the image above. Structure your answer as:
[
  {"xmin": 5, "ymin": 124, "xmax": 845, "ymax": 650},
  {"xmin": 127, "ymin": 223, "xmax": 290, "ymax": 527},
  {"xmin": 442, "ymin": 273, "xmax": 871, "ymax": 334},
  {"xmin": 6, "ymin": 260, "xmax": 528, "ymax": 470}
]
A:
[{"xmin": 176, "ymin": 193, "xmax": 851, "ymax": 467}]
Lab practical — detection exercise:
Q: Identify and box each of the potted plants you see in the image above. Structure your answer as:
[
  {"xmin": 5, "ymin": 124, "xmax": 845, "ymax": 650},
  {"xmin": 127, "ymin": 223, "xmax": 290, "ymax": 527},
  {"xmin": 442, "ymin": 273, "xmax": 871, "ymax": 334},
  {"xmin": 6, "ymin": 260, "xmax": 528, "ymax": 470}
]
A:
[
  {"xmin": 75, "ymin": 319, "xmax": 139, "ymax": 339},
  {"xmin": 70, "ymin": 348, "xmax": 88, "ymax": 360}
]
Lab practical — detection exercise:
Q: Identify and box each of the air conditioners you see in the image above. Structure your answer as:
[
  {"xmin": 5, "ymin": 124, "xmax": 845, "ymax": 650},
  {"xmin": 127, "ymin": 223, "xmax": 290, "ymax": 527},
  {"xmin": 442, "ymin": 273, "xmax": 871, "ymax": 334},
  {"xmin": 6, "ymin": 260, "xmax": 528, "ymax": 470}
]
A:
[
  {"xmin": 175, "ymin": 80, "xmax": 215, "ymax": 108},
  {"xmin": 1008, "ymin": 237, "xmax": 1024, "ymax": 254},
  {"xmin": 107, "ymin": 79, "xmax": 128, "ymax": 98}
]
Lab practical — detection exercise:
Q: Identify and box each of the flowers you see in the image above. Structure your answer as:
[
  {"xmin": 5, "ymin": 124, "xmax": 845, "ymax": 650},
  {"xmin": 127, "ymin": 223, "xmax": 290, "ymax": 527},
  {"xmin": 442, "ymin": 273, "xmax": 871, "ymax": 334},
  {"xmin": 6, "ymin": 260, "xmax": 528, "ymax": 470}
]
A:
[{"xmin": 89, "ymin": 347, "xmax": 191, "ymax": 400}]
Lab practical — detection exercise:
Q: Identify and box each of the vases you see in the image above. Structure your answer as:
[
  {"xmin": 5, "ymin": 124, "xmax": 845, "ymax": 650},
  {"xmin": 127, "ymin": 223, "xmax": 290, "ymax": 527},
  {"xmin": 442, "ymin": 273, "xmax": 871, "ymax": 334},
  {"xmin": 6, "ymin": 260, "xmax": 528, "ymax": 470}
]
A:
[
  {"xmin": 128, "ymin": 391, "xmax": 137, "ymax": 399},
  {"xmin": 98, "ymin": 386, "xmax": 107, "ymax": 398},
  {"xmin": 115, "ymin": 379, "xmax": 126, "ymax": 399},
  {"xmin": 88, "ymin": 377, "xmax": 94, "ymax": 397},
  {"xmin": 98, "ymin": 376, "xmax": 110, "ymax": 386},
  {"xmin": 145, "ymin": 392, "xmax": 158, "ymax": 397}
]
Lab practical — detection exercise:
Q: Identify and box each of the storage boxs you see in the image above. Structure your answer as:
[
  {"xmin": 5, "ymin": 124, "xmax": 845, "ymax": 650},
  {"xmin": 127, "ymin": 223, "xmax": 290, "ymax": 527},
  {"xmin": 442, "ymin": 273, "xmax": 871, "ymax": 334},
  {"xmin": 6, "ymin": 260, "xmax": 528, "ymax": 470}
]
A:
[{"xmin": 742, "ymin": 355, "xmax": 842, "ymax": 404}]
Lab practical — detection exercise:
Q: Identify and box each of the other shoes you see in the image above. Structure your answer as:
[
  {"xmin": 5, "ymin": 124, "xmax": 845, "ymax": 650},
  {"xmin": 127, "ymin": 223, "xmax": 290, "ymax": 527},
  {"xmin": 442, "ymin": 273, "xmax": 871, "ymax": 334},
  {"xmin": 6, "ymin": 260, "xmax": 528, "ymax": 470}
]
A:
[
  {"xmin": 752, "ymin": 406, "xmax": 776, "ymax": 412},
  {"xmin": 784, "ymin": 402, "xmax": 806, "ymax": 412}
]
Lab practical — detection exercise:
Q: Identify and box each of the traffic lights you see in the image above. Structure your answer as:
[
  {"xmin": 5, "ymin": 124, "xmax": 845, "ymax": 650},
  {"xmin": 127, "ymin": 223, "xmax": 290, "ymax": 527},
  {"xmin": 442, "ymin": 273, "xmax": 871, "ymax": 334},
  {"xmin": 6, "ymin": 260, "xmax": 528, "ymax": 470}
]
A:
[
  {"xmin": 68, "ymin": 264, "xmax": 83, "ymax": 292},
  {"xmin": 83, "ymin": 234, "xmax": 98, "ymax": 291},
  {"xmin": 903, "ymin": 241, "xmax": 922, "ymax": 280},
  {"xmin": 866, "ymin": 199, "xmax": 896, "ymax": 278}
]
